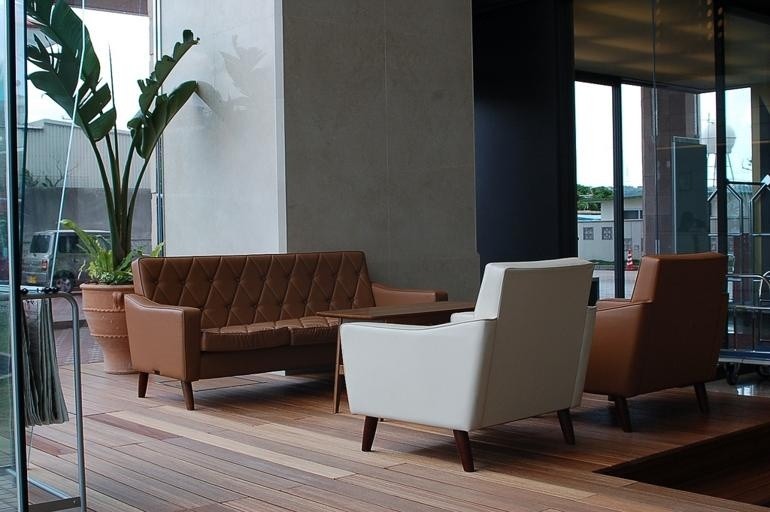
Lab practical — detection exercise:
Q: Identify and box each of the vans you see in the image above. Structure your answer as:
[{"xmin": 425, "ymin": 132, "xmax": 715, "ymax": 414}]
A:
[{"xmin": 23, "ymin": 229, "xmax": 112, "ymax": 292}]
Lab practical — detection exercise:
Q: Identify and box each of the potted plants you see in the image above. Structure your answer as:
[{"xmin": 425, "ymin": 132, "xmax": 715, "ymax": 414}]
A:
[{"xmin": 23, "ymin": 1, "xmax": 200, "ymax": 378}]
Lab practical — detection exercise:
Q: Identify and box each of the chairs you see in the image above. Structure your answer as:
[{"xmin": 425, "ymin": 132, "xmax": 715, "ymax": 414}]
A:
[
  {"xmin": 340, "ymin": 255, "xmax": 599, "ymax": 473},
  {"xmin": 583, "ymin": 251, "xmax": 732, "ymax": 431}
]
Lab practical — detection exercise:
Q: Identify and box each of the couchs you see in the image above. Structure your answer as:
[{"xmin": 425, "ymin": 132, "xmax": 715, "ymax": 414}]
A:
[{"xmin": 121, "ymin": 248, "xmax": 447, "ymax": 413}]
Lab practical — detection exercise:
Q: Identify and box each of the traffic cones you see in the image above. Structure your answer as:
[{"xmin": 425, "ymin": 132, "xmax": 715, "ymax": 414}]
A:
[{"xmin": 624, "ymin": 248, "xmax": 638, "ymax": 271}]
